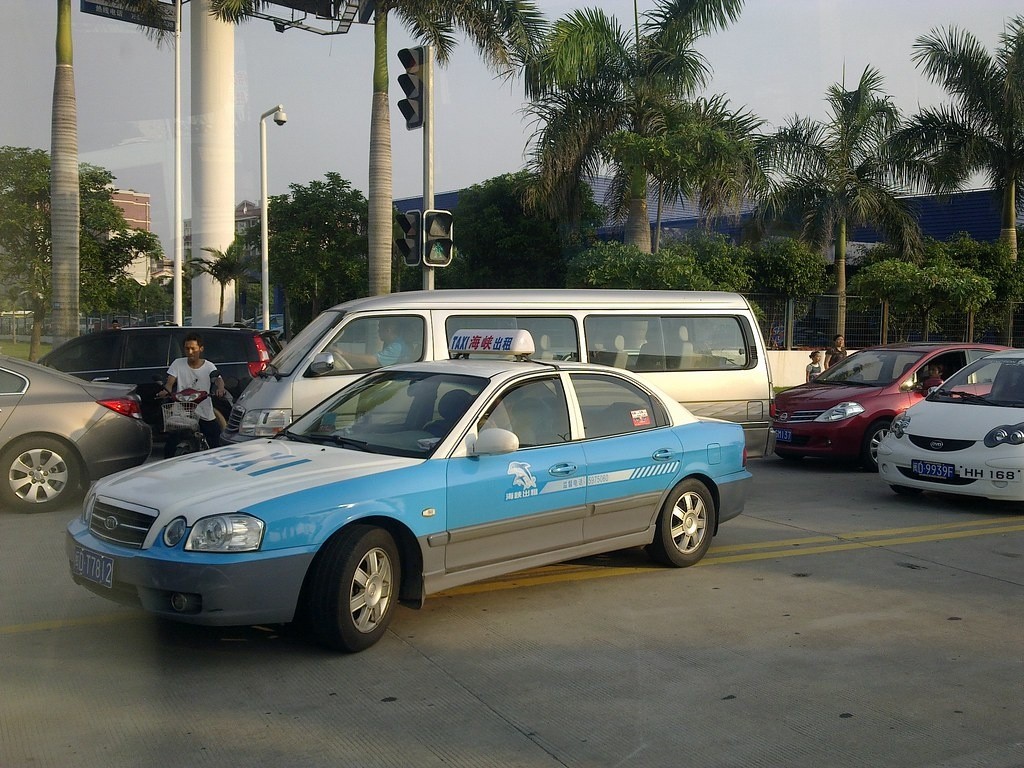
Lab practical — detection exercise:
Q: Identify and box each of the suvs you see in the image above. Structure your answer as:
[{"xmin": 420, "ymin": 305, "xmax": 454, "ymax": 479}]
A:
[{"xmin": 768, "ymin": 341, "xmax": 1015, "ymax": 471}]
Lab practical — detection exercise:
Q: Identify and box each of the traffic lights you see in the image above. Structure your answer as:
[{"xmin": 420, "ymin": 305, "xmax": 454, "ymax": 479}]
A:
[
  {"xmin": 397, "ymin": 45, "xmax": 427, "ymax": 131},
  {"xmin": 395, "ymin": 210, "xmax": 421, "ymax": 268},
  {"xmin": 422, "ymin": 210, "xmax": 454, "ymax": 267}
]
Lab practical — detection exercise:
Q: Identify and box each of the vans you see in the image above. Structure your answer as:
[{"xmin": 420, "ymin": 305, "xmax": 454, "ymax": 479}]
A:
[
  {"xmin": 37, "ymin": 325, "xmax": 283, "ymax": 446},
  {"xmin": 216, "ymin": 287, "xmax": 782, "ymax": 462}
]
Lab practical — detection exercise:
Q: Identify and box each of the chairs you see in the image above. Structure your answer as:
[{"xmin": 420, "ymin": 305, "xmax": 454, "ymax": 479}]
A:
[
  {"xmin": 532, "ymin": 334, "xmax": 551, "ymax": 361},
  {"xmin": 423, "ymin": 389, "xmax": 475, "ymax": 436},
  {"xmin": 666, "ymin": 325, "xmax": 694, "ymax": 371},
  {"xmin": 593, "ymin": 335, "xmax": 628, "ymax": 369}
]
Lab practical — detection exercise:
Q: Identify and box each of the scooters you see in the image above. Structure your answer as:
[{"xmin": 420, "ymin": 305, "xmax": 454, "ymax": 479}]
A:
[{"xmin": 152, "ymin": 369, "xmax": 227, "ymax": 460}]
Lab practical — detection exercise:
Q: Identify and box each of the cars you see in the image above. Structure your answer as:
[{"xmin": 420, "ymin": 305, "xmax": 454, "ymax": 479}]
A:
[
  {"xmin": 63, "ymin": 328, "xmax": 754, "ymax": 654},
  {"xmin": 0, "ymin": 353, "xmax": 153, "ymax": 514},
  {"xmin": 876, "ymin": 348, "xmax": 1024, "ymax": 501}
]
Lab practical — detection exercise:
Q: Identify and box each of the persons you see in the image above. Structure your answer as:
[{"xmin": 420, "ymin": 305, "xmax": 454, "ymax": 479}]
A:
[
  {"xmin": 806, "ymin": 351, "xmax": 823, "ymax": 384},
  {"xmin": 330, "ymin": 319, "xmax": 410, "ymax": 369},
  {"xmin": 478, "ymin": 386, "xmax": 536, "ymax": 446},
  {"xmin": 112, "ymin": 320, "xmax": 118, "ymax": 329},
  {"xmin": 913, "ymin": 364, "xmax": 943, "ymax": 390},
  {"xmin": 157, "ymin": 333, "xmax": 225, "ymax": 459},
  {"xmin": 824, "ymin": 334, "xmax": 847, "ymax": 370}
]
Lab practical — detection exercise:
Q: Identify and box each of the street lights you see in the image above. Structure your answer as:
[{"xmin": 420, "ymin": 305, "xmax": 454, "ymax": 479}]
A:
[{"xmin": 260, "ymin": 104, "xmax": 288, "ymax": 330}]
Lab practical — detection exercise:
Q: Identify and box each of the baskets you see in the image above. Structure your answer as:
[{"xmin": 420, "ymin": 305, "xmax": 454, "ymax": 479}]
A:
[{"xmin": 161, "ymin": 401, "xmax": 203, "ymax": 434}]
